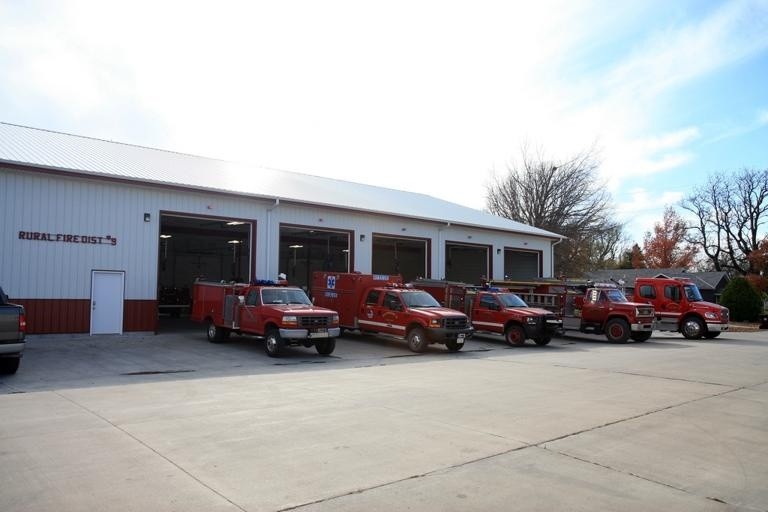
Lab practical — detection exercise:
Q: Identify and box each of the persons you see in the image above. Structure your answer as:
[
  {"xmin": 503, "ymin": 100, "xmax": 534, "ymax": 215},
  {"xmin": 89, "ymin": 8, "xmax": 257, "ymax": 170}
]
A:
[
  {"xmin": 276, "ymin": 272, "xmax": 288, "ymax": 287},
  {"xmin": 582, "ymin": 279, "xmax": 596, "ymax": 294}
]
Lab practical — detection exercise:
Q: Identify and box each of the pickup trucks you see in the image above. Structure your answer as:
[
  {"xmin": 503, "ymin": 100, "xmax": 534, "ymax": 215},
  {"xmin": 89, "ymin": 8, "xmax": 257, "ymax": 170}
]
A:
[{"xmin": 0, "ymin": 280, "xmax": 28, "ymax": 374}]
